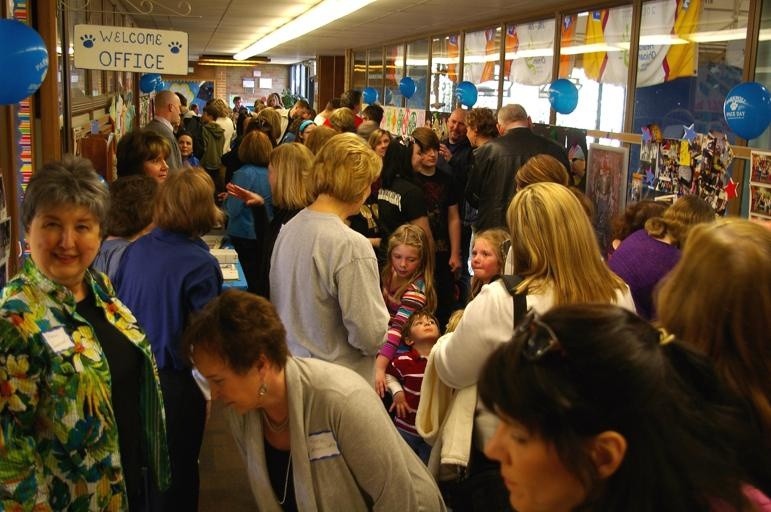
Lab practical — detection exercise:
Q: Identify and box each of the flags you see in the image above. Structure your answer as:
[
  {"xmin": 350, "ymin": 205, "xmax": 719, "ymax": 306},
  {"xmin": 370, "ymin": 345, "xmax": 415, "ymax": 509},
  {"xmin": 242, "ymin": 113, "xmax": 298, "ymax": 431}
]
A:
[
  {"xmin": 584, "ymin": 0, "xmax": 699, "ymax": 88},
  {"xmin": 505, "ymin": 13, "xmax": 575, "ymax": 83},
  {"xmin": 448, "ymin": 29, "xmax": 494, "ymax": 83},
  {"xmin": 390, "ymin": 42, "xmax": 411, "ymax": 84}
]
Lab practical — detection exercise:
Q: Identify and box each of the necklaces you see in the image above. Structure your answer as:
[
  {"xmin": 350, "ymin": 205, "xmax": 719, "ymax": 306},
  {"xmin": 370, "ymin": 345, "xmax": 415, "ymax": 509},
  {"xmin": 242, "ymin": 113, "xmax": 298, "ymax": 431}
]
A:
[
  {"xmin": 260, "ymin": 410, "xmax": 288, "ymax": 433},
  {"xmin": 278, "ymin": 447, "xmax": 289, "ymax": 506}
]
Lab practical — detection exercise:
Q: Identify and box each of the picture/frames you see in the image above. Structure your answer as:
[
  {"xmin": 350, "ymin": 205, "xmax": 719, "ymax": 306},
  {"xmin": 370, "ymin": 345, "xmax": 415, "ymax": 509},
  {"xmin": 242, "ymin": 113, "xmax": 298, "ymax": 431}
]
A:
[{"xmin": 585, "ymin": 142, "xmax": 630, "ymax": 256}]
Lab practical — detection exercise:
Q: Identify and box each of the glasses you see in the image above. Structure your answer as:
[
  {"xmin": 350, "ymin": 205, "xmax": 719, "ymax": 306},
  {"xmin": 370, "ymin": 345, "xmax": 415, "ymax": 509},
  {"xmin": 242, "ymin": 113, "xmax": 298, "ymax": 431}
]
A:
[{"xmin": 513, "ymin": 307, "xmax": 566, "ymax": 364}]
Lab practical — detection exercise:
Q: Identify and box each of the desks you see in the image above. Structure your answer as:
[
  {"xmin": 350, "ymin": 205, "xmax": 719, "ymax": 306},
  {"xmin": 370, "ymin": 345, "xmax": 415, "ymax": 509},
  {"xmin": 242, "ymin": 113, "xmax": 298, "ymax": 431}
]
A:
[{"xmin": 218, "ymin": 242, "xmax": 249, "ymax": 292}]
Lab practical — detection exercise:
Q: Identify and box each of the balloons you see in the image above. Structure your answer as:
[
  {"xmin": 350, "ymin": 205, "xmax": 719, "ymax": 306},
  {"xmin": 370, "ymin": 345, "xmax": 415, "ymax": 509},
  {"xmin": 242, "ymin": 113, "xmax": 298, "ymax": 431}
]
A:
[
  {"xmin": 399, "ymin": 76, "xmax": 414, "ymax": 100},
  {"xmin": 141, "ymin": 74, "xmax": 163, "ymax": 93},
  {"xmin": 549, "ymin": 79, "xmax": 581, "ymax": 114},
  {"xmin": 455, "ymin": 81, "xmax": 477, "ymax": 106},
  {"xmin": 724, "ymin": 81, "xmax": 769, "ymax": 141},
  {"xmin": 0, "ymin": 18, "xmax": 50, "ymax": 104}
]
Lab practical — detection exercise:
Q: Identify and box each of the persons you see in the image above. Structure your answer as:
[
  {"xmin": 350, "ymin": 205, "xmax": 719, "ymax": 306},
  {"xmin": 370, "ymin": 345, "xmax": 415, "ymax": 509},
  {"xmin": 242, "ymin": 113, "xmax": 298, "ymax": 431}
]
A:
[
  {"xmin": 0, "ymin": 156, "xmax": 174, "ymax": 511},
  {"xmin": 478, "ymin": 303, "xmax": 770, "ymax": 511},
  {"xmin": 415, "ymin": 182, "xmax": 639, "ymax": 512},
  {"xmin": 181, "ymin": 289, "xmax": 448, "ymax": 512},
  {"xmin": 89, "ymin": 86, "xmax": 769, "ymax": 466},
  {"xmin": 114, "ymin": 165, "xmax": 223, "ymax": 512}
]
